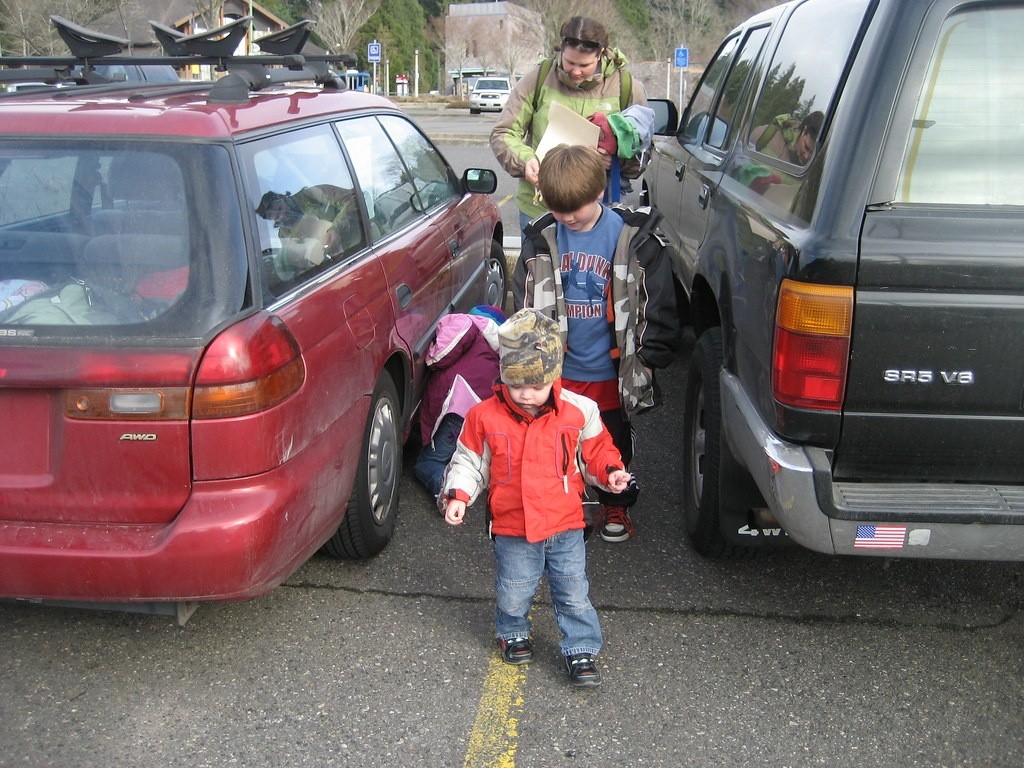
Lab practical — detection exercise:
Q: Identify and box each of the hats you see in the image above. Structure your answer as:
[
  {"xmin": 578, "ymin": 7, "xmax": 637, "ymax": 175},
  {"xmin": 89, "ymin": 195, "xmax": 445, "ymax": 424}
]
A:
[
  {"xmin": 469, "ymin": 305, "xmax": 506, "ymax": 325},
  {"xmin": 498, "ymin": 307, "xmax": 562, "ymax": 386}
]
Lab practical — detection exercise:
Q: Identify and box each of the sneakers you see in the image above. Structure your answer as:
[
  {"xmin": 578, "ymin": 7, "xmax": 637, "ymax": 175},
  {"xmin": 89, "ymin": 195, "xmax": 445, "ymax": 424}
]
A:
[
  {"xmin": 564, "ymin": 652, "xmax": 602, "ymax": 686},
  {"xmin": 499, "ymin": 637, "xmax": 533, "ymax": 665},
  {"xmin": 593, "ymin": 504, "xmax": 634, "ymax": 542}
]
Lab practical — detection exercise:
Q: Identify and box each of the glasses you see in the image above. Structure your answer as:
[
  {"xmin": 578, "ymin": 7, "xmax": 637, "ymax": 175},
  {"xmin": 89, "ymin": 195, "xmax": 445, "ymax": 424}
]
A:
[{"xmin": 562, "ymin": 37, "xmax": 602, "ymax": 56}]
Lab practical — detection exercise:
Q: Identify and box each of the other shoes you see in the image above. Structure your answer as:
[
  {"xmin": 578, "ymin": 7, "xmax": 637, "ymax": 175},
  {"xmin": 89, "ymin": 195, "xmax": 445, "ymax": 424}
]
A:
[{"xmin": 435, "ymin": 462, "xmax": 459, "ymax": 519}]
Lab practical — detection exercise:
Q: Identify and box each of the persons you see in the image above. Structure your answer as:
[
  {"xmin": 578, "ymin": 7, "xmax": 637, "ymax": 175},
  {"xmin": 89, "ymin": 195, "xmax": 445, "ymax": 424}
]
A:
[
  {"xmin": 513, "ymin": 143, "xmax": 680, "ymax": 542},
  {"xmin": 255, "ymin": 184, "xmax": 383, "ymax": 276},
  {"xmin": 489, "ymin": 15, "xmax": 655, "ymax": 255},
  {"xmin": 698, "ymin": 111, "xmax": 829, "ymax": 396},
  {"xmin": 413, "ymin": 305, "xmax": 510, "ymax": 515},
  {"xmin": 435, "ymin": 308, "xmax": 631, "ymax": 686}
]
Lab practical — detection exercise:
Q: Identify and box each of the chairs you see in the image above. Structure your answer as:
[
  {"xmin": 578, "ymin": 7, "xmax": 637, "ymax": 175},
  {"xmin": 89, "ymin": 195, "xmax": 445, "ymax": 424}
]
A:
[{"xmin": 91, "ymin": 151, "xmax": 185, "ymax": 235}]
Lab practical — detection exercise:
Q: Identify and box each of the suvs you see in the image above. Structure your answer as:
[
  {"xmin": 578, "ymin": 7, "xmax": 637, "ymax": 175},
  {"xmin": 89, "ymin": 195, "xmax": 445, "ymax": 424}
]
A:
[
  {"xmin": 638, "ymin": 1, "xmax": 1023, "ymax": 563},
  {"xmin": 468, "ymin": 75, "xmax": 512, "ymax": 115},
  {"xmin": 0, "ymin": 16, "xmax": 506, "ymax": 623}
]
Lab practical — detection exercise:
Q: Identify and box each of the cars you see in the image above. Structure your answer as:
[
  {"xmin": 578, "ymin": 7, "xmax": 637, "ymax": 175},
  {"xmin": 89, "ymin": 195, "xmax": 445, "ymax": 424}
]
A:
[{"xmin": 5, "ymin": 82, "xmax": 76, "ymax": 93}]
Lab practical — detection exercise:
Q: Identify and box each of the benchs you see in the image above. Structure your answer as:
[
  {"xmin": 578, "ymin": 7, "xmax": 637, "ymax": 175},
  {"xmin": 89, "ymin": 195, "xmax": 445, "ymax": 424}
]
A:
[{"xmin": 0, "ymin": 230, "xmax": 188, "ymax": 289}]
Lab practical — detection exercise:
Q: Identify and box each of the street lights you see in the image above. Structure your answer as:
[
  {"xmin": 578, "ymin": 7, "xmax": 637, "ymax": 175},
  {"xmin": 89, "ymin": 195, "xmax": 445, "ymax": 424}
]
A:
[{"xmin": 326, "ymin": 43, "xmax": 340, "ymax": 58}]
